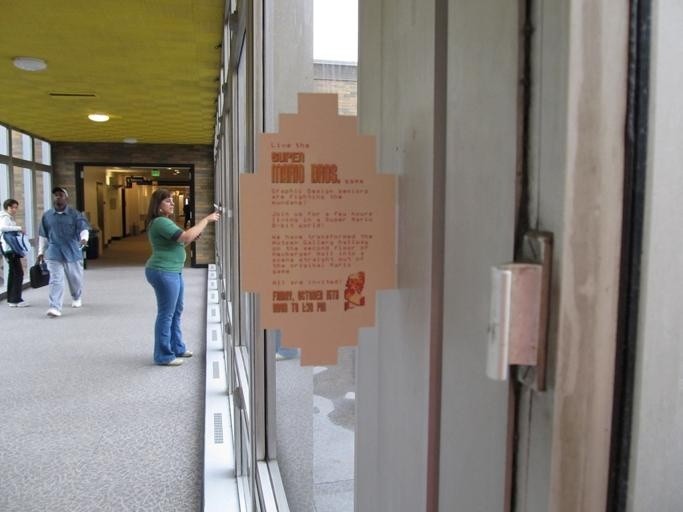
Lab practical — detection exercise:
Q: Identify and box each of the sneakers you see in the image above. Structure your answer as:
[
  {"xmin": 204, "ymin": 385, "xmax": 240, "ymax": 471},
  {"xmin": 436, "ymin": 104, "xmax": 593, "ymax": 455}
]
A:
[
  {"xmin": 71, "ymin": 297, "xmax": 81, "ymax": 308},
  {"xmin": 9, "ymin": 302, "xmax": 30, "ymax": 308},
  {"xmin": 181, "ymin": 351, "xmax": 193, "ymax": 357},
  {"xmin": 169, "ymin": 359, "xmax": 183, "ymax": 366},
  {"xmin": 46, "ymin": 309, "xmax": 61, "ymax": 317}
]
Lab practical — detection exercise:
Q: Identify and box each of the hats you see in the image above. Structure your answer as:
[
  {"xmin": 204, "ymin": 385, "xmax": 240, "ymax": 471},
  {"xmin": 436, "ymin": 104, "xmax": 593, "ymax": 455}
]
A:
[{"xmin": 51, "ymin": 187, "xmax": 69, "ymax": 197}]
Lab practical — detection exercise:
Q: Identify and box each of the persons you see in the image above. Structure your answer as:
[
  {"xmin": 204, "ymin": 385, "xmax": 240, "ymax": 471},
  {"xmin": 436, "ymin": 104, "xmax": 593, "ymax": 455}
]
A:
[
  {"xmin": 36, "ymin": 187, "xmax": 89, "ymax": 316},
  {"xmin": 184, "ymin": 201, "xmax": 191, "ymax": 228},
  {"xmin": 145, "ymin": 189, "xmax": 221, "ymax": 366},
  {"xmin": 0, "ymin": 199, "xmax": 29, "ymax": 307}
]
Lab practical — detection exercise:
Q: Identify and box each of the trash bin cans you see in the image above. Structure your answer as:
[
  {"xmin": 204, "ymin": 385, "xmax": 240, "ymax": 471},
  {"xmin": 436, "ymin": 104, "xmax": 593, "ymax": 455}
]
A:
[
  {"xmin": 132, "ymin": 224, "xmax": 139, "ymax": 236},
  {"xmin": 86, "ymin": 230, "xmax": 99, "ymax": 259}
]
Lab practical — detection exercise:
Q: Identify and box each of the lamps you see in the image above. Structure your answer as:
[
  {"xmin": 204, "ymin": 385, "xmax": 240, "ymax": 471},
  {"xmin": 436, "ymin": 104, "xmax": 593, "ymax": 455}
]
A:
[
  {"xmin": 13, "ymin": 56, "xmax": 48, "ymax": 71},
  {"xmin": 87, "ymin": 114, "xmax": 110, "ymax": 122}
]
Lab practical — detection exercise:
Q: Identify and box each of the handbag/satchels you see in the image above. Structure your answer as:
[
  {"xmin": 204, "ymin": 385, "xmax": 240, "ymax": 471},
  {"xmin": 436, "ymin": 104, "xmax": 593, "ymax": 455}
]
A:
[
  {"xmin": 0, "ymin": 230, "xmax": 31, "ymax": 257},
  {"xmin": 30, "ymin": 255, "xmax": 49, "ymax": 288}
]
